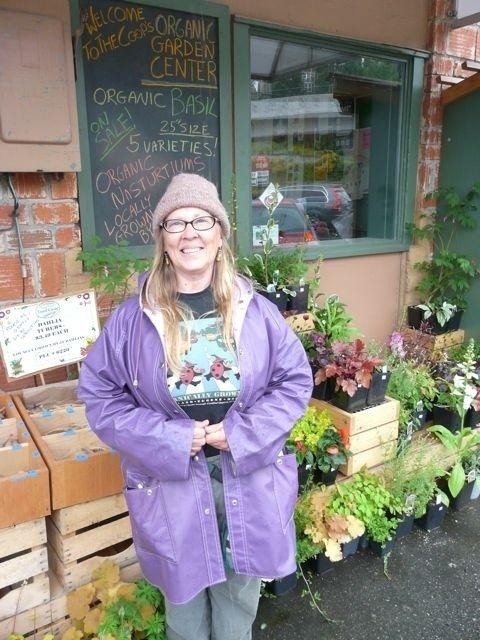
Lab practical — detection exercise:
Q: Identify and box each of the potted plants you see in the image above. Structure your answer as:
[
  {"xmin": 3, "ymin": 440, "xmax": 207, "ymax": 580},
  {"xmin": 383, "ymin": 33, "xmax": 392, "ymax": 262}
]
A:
[
  {"xmin": 99, "ymin": 580, "xmax": 167, "ymax": 640},
  {"xmin": 239, "ymin": 185, "xmax": 480, "ymax": 622}
]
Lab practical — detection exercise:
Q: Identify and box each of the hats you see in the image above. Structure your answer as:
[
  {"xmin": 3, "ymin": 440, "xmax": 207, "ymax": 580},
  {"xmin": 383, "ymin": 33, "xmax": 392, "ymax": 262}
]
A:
[{"xmin": 151, "ymin": 174, "xmax": 230, "ymax": 240}]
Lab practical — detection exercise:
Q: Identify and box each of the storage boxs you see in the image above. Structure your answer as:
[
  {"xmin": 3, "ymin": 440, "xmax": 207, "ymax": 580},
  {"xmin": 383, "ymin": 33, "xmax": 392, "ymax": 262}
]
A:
[
  {"xmin": 0, "ymin": 391, "xmax": 52, "ymax": 526},
  {"xmin": 10, "ymin": 381, "xmax": 126, "ymax": 510}
]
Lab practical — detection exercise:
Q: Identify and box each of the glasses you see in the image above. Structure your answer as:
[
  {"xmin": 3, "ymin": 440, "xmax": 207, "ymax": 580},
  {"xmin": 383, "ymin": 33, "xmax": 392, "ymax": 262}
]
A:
[{"xmin": 159, "ymin": 215, "xmax": 220, "ymax": 231}]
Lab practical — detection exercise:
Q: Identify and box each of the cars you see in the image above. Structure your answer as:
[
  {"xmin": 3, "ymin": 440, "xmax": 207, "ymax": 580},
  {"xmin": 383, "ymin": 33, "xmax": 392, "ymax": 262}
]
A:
[{"xmin": 252, "ymin": 199, "xmax": 318, "ymax": 245}]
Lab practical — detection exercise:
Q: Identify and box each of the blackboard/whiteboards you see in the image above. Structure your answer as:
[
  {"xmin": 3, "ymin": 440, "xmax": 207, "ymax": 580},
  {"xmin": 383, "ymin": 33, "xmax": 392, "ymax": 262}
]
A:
[{"xmin": 69, "ymin": 0, "xmax": 234, "ymax": 269}]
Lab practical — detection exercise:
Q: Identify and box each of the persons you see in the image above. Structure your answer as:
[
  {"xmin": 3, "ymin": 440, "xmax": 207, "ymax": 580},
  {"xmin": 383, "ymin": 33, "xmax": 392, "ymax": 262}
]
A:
[{"xmin": 78, "ymin": 174, "xmax": 312, "ymax": 640}]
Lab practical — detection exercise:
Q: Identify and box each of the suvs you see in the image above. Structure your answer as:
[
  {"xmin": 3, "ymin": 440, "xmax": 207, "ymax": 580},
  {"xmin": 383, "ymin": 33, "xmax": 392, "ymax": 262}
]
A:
[{"xmin": 270, "ymin": 183, "xmax": 353, "ymax": 241}]
